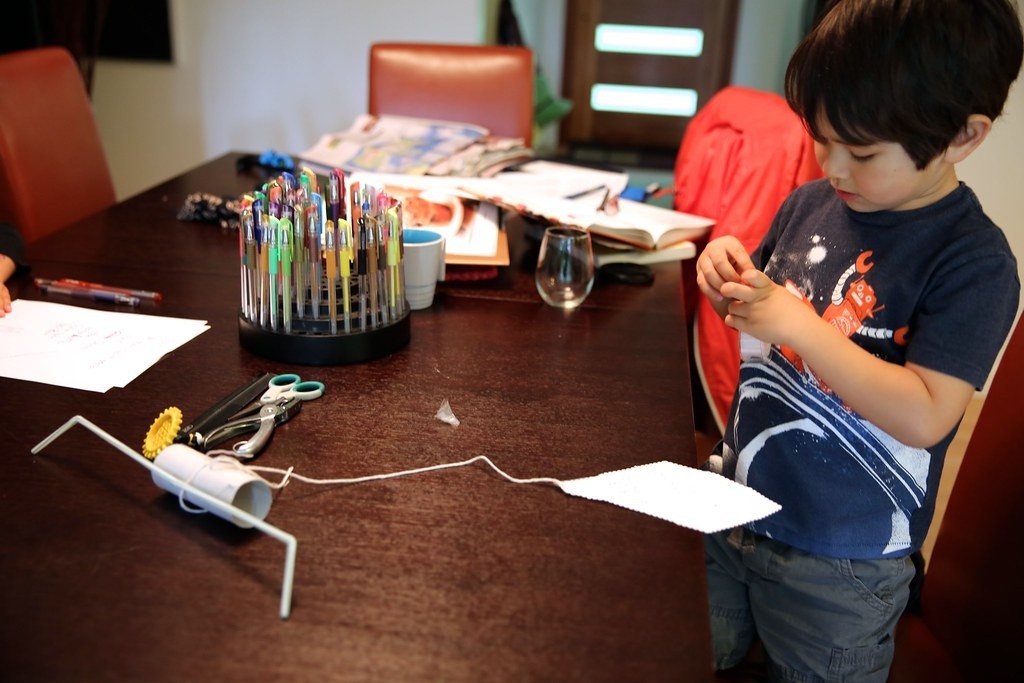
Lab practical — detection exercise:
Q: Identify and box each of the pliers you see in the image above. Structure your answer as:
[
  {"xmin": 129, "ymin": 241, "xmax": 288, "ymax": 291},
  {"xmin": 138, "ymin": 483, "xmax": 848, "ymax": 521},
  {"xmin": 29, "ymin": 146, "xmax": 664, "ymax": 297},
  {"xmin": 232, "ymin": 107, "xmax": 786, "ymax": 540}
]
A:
[{"xmin": 195, "ymin": 395, "xmax": 303, "ymax": 457}]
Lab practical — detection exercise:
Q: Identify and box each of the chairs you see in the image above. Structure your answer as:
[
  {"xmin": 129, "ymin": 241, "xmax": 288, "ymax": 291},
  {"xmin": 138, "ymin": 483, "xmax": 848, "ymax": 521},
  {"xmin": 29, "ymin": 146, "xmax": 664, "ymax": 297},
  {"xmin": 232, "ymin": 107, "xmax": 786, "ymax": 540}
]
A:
[
  {"xmin": 0, "ymin": 47, "xmax": 117, "ymax": 246},
  {"xmin": 368, "ymin": 43, "xmax": 532, "ymax": 153},
  {"xmin": 553, "ymin": 0, "xmax": 739, "ymax": 174}
]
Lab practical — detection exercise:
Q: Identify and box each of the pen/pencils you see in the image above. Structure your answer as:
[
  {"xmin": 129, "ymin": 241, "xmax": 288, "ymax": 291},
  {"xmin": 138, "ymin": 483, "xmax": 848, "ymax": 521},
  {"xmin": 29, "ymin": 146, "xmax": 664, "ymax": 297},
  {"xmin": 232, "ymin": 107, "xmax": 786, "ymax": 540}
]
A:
[
  {"xmin": 33, "ymin": 276, "xmax": 162, "ymax": 307},
  {"xmin": 565, "ymin": 183, "xmax": 610, "ymax": 210},
  {"xmin": 239, "ymin": 166, "xmax": 405, "ymax": 336}
]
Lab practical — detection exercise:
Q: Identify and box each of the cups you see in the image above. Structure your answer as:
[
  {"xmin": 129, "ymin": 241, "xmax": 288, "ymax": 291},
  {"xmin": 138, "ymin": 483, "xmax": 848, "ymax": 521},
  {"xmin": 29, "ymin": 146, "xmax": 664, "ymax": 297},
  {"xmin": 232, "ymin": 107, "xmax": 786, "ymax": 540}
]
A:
[
  {"xmin": 402, "ymin": 227, "xmax": 445, "ymax": 310},
  {"xmin": 535, "ymin": 225, "xmax": 596, "ymax": 312}
]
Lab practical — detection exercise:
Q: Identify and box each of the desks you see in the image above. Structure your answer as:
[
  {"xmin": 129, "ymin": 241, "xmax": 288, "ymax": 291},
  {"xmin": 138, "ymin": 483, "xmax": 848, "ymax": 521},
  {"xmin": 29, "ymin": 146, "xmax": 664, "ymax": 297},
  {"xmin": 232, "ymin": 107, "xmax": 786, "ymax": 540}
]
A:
[{"xmin": 0, "ymin": 151, "xmax": 715, "ymax": 683}]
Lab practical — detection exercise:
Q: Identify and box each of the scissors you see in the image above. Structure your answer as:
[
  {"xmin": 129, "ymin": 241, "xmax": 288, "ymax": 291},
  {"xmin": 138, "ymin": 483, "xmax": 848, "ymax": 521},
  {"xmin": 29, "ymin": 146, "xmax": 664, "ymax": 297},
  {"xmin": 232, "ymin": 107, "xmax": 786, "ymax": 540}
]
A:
[{"xmin": 228, "ymin": 373, "xmax": 326, "ymax": 420}]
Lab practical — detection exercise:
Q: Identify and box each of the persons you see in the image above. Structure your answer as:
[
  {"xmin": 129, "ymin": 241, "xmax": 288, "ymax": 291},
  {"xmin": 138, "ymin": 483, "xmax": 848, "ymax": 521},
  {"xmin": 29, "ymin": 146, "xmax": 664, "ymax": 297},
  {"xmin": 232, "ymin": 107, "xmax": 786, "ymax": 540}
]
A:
[{"xmin": 695, "ymin": 0, "xmax": 1024, "ymax": 683}]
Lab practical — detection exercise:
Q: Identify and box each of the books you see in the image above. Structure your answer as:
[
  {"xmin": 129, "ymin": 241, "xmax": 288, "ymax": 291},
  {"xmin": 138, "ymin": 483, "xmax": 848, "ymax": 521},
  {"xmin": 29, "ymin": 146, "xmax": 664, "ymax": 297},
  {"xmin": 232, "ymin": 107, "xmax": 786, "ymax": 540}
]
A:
[{"xmin": 448, "ymin": 173, "xmax": 716, "ymax": 268}]
